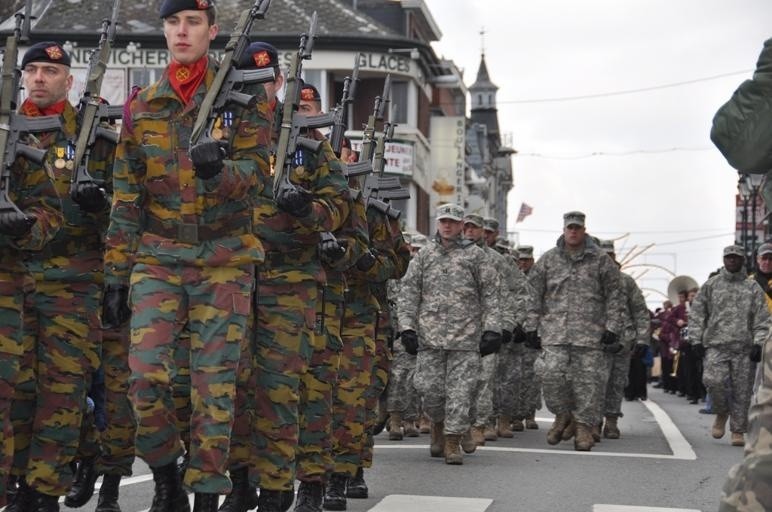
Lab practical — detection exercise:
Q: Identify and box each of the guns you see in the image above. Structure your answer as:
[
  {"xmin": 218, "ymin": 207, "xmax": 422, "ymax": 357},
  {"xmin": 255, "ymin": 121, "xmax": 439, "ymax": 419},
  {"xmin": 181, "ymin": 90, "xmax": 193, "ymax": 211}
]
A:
[
  {"xmin": 273, "ymin": 12, "xmax": 411, "ymax": 218},
  {"xmin": 0, "ymin": 1, "xmax": 61, "ymax": 222},
  {"xmin": 189, "ymin": 0, "xmax": 275, "ymax": 160},
  {"xmin": 69, "ymin": 0, "xmax": 126, "ymax": 195}
]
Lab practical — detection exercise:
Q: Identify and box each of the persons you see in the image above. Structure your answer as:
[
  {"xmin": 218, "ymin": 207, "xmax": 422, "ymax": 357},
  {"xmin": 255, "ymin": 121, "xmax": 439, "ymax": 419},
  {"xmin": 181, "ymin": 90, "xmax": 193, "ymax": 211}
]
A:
[
  {"xmin": 101, "ymin": 0, "xmax": 275, "ymax": 512},
  {"xmin": 709, "ymin": 37, "xmax": 771, "ymax": 512},
  {"xmin": 0, "ymin": 43, "xmax": 112, "ymax": 511}
]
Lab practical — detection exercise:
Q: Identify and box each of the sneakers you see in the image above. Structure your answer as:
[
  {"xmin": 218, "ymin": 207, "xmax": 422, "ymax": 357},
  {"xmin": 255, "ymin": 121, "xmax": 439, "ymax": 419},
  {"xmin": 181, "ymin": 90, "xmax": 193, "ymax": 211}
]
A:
[{"xmin": 630, "ymin": 383, "xmax": 710, "ymax": 415}]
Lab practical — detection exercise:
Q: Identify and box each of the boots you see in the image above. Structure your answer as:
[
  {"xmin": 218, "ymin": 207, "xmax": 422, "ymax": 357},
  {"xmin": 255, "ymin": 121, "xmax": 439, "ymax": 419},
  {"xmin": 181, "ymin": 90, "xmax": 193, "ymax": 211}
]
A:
[
  {"xmin": 345, "ymin": 466, "xmax": 369, "ymax": 500},
  {"xmin": 293, "ymin": 480, "xmax": 324, "ymax": 512},
  {"xmin": 710, "ymin": 411, "xmax": 730, "ymax": 439},
  {"xmin": 192, "ymin": 492, "xmax": 219, "ymax": 512},
  {"xmin": 471, "ymin": 422, "xmax": 488, "ymax": 447},
  {"xmin": 496, "ymin": 415, "xmax": 514, "ymax": 437},
  {"xmin": 443, "ymin": 432, "xmax": 464, "ymax": 465},
  {"xmin": 147, "ymin": 478, "xmax": 191, "ymax": 512},
  {"xmin": 481, "ymin": 419, "xmax": 498, "ymax": 442},
  {"xmin": 459, "ymin": 430, "xmax": 478, "ymax": 455},
  {"xmin": 512, "ymin": 418, "xmax": 525, "ymax": 432},
  {"xmin": 524, "ymin": 412, "xmax": 538, "ymax": 430},
  {"xmin": 430, "ymin": 421, "xmax": 445, "ymax": 456},
  {"xmin": 219, "ymin": 470, "xmax": 295, "ymax": 512},
  {"xmin": 603, "ymin": 415, "xmax": 621, "ymax": 439},
  {"xmin": 419, "ymin": 417, "xmax": 434, "ymax": 436},
  {"xmin": 64, "ymin": 456, "xmax": 96, "ymax": 507},
  {"xmin": 575, "ymin": 424, "xmax": 594, "ymax": 451},
  {"xmin": 403, "ymin": 420, "xmax": 422, "ymax": 438},
  {"xmin": 94, "ymin": 472, "xmax": 121, "ymax": 512},
  {"xmin": 731, "ymin": 431, "xmax": 746, "ymax": 447},
  {"xmin": 322, "ymin": 472, "xmax": 349, "ymax": 511},
  {"xmin": 561, "ymin": 421, "xmax": 576, "ymax": 440},
  {"xmin": 592, "ymin": 425, "xmax": 602, "ymax": 443},
  {"xmin": 546, "ymin": 412, "xmax": 572, "ymax": 447},
  {"xmin": 388, "ymin": 415, "xmax": 404, "ymax": 444},
  {"xmin": 2, "ymin": 474, "xmax": 60, "ymax": 512}
]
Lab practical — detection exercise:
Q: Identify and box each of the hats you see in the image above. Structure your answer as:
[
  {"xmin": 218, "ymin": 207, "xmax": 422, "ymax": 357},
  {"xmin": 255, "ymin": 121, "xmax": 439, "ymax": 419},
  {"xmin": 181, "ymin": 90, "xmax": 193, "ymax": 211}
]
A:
[
  {"xmin": 411, "ymin": 234, "xmax": 429, "ymax": 249},
  {"xmin": 433, "ymin": 203, "xmax": 464, "ymax": 222},
  {"xmin": 298, "ymin": 83, "xmax": 321, "ymax": 101},
  {"xmin": 592, "ymin": 236, "xmax": 615, "ymax": 256},
  {"xmin": 157, "ymin": 0, "xmax": 215, "ymax": 20},
  {"xmin": 340, "ymin": 134, "xmax": 352, "ymax": 151},
  {"xmin": 482, "ymin": 217, "xmax": 500, "ymax": 232},
  {"xmin": 461, "ymin": 214, "xmax": 485, "ymax": 228},
  {"xmin": 518, "ymin": 245, "xmax": 533, "ymax": 260},
  {"xmin": 496, "ymin": 236, "xmax": 521, "ymax": 260},
  {"xmin": 400, "ymin": 230, "xmax": 411, "ymax": 244},
  {"xmin": 81, "ymin": 96, "xmax": 114, "ymax": 124},
  {"xmin": 562, "ymin": 210, "xmax": 585, "ymax": 229},
  {"xmin": 723, "ymin": 244, "xmax": 745, "ymax": 257},
  {"xmin": 20, "ymin": 41, "xmax": 71, "ymax": 72},
  {"xmin": 234, "ymin": 41, "xmax": 280, "ymax": 79}
]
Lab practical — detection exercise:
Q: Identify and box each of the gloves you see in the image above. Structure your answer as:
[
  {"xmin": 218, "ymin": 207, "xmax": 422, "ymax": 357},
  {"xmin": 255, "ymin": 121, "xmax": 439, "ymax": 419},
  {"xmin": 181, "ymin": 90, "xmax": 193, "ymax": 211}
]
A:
[
  {"xmin": 70, "ymin": 176, "xmax": 108, "ymax": 211},
  {"xmin": 524, "ymin": 330, "xmax": 548, "ymax": 353},
  {"xmin": 318, "ymin": 237, "xmax": 347, "ymax": 260},
  {"xmin": 499, "ymin": 327, "xmax": 515, "ymax": 344},
  {"xmin": 599, "ymin": 329, "xmax": 625, "ymax": 358},
  {"xmin": 478, "ymin": 329, "xmax": 501, "ymax": 358},
  {"xmin": 399, "ymin": 329, "xmax": 419, "ymax": 356},
  {"xmin": 748, "ymin": 344, "xmax": 763, "ymax": 362},
  {"xmin": 633, "ymin": 341, "xmax": 649, "ymax": 361},
  {"xmin": 103, "ymin": 284, "xmax": 133, "ymax": 325},
  {"xmin": 0, "ymin": 208, "xmax": 38, "ymax": 238},
  {"xmin": 191, "ymin": 141, "xmax": 226, "ymax": 178},
  {"xmin": 691, "ymin": 343, "xmax": 706, "ymax": 359},
  {"xmin": 757, "ymin": 242, "xmax": 772, "ymax": 256},
  {"xmin": 513, "ymin": 324, "xmax": 528, "ymax": 342},
  {"xmin": 279, "ymin": 184, "xmax": 314, "ymax": 214}
]
